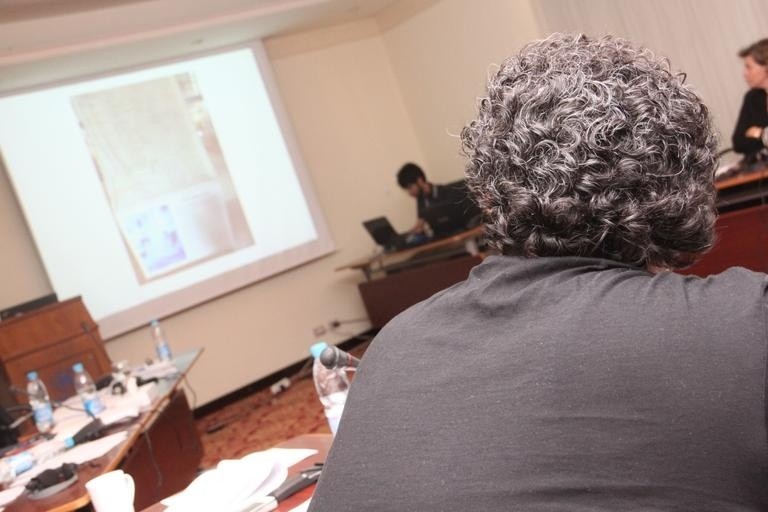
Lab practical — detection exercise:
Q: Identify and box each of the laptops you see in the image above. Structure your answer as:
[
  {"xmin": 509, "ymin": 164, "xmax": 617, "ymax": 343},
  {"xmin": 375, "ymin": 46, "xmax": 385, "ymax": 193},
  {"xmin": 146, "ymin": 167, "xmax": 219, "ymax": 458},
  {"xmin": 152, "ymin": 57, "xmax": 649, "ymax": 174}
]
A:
[{"xmin": 363, "ymin": 216, "xmax": 404, "ymax": 251}]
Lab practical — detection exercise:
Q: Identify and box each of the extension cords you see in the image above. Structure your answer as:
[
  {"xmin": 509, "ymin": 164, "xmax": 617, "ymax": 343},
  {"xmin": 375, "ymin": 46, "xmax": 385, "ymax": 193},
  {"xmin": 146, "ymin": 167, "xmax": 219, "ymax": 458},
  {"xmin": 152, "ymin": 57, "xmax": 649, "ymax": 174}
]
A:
[{"xmin": 270, "ymin": 377, "xmax": 291, "ymax": 395}]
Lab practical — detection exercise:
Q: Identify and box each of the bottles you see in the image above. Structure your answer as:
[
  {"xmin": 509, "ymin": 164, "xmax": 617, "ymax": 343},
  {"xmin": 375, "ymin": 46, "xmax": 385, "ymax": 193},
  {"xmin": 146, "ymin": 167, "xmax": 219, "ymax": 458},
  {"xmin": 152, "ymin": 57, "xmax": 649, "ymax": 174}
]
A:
[
  {"xmin": 6, "ymin": 437, "xmax": 76, "ymax": 476},
  {"xmin": 310, "ymin": 343, "xmax": 350, "ymax": 437},
  {"xmin": 149, "ymin": 319, "xmax": 177, "ymax": 362},
  {"xmin": 24, "ymin": 371, "xmax": 55, "ymax": 434},
  {"xmin": 71, "ymin": 362, "xmax": 107, "ymax": 420}
]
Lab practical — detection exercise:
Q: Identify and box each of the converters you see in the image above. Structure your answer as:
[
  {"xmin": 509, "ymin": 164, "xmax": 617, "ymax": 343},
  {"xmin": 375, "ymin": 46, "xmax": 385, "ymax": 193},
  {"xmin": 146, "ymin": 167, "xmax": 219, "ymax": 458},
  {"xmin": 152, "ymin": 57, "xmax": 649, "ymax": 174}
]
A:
[
  {"xmin": 334, "ymin": 322, "xmax": 341, "ymax": 327},
  {"xmin": 206, "ymin": 422, "xmax": 225, "ymax": 432}
]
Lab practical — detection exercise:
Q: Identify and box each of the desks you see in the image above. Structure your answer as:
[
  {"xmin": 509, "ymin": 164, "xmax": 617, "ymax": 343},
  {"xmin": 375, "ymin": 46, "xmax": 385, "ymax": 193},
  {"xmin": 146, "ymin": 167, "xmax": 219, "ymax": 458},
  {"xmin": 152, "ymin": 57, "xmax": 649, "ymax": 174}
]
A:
[
  {"xmin": 0, "ymin": 349, "xmax": 332, "ymax": 512},
  {"xmin": 345, "ymin": 168, "xmax": 768, "ymax": 331}
]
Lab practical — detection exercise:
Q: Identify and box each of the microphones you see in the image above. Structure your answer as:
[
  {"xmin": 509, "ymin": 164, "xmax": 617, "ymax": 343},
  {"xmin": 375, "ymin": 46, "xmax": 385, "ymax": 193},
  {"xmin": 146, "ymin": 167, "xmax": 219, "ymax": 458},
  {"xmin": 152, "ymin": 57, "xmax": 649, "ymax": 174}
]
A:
[
  {"xmin": 319, "ymin": 346, "xmax": 361, "ymax": 371},
  {"xmin": 7, "ymin": 386, "xmax": 105, "ymax": 443},
  {"xmin": 80, "ymin": 321, "xmax": 119, "ymax": 390}
]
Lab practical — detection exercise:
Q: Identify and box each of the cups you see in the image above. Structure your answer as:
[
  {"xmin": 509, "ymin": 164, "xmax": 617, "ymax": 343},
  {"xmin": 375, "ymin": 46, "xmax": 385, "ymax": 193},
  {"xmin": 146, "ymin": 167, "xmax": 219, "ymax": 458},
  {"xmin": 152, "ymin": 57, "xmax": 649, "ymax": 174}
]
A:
[{"xmin": 83, "ymin": 469, "xmax": 136, "ymax": 512}]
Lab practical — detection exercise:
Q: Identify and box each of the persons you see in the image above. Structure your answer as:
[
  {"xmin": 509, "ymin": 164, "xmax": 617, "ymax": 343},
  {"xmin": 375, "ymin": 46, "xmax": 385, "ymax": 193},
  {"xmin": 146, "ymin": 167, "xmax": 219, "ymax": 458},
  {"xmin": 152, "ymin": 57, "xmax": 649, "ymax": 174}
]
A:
[
  {"xmin": 728, "ymin": 36, "xmax": 768, "ymax": 166},
  {"xmin": 393, "ymin": 161, "xmax": 482, "ymax": 240},
  {"xmin": 304, "ymin": 28, "xmax": 766, "ymax": 510}
]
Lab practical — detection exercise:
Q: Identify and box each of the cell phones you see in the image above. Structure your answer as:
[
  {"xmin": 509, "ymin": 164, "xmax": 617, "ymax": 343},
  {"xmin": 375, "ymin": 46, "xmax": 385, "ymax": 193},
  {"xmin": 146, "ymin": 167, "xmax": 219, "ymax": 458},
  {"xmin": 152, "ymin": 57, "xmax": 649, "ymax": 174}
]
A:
[{"xmin": 242, "ymin": 494, "xmax": 279, "ymax": 512}]
